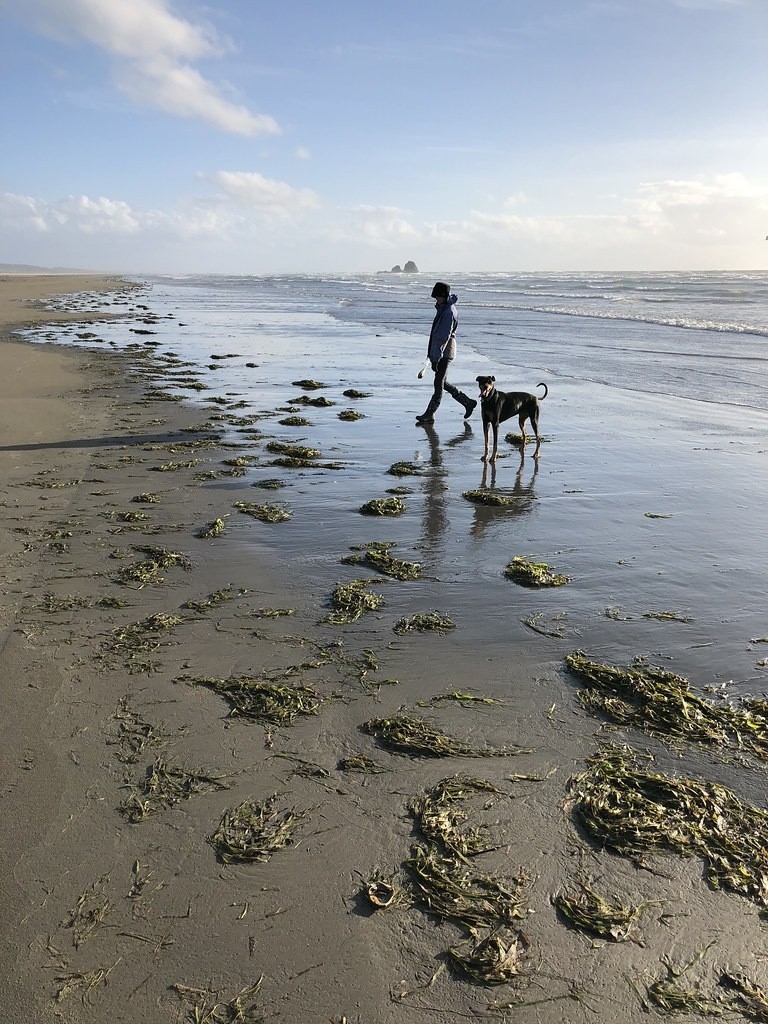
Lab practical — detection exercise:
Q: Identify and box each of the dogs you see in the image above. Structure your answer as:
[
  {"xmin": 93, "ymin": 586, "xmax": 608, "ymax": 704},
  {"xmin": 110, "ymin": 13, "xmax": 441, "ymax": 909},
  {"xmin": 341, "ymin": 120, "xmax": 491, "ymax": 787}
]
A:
[{"xmin": 476, "ymin": 375, "xmax": 548, "ymax": 462}]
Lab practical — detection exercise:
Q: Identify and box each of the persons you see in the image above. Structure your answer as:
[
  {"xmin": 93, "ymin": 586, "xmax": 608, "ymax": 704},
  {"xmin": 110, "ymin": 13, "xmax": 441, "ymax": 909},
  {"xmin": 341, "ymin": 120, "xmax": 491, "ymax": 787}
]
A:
[{"xmin": 416, "ymin": 282, "xmax": 477, "ymax": 423}]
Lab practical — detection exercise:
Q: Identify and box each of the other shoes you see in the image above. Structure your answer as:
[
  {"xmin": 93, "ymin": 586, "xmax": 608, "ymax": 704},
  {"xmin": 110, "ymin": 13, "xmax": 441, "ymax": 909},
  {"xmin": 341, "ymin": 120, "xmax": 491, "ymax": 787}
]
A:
[
  {"xmin": 463, "ymin": 421, "xmax": 474, "ymax": 440},
  {"xmin": 464, "ymin": 400, "xmax": 477, "ymax": 419},
  {"xmin": 416, "ymin": 413, "xmax": 434, "ymax": 422},
  {"xmin": 415, "ymin": 421, "xmax": 434, "ymax": 427}
]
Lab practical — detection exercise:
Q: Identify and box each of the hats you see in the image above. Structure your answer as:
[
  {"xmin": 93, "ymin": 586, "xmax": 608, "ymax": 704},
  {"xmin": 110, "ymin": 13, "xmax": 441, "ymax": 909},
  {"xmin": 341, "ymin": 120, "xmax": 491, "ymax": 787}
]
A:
[{"xmin": 430, "ymin": 281, "xmax": 450, "ymax": 298}]
